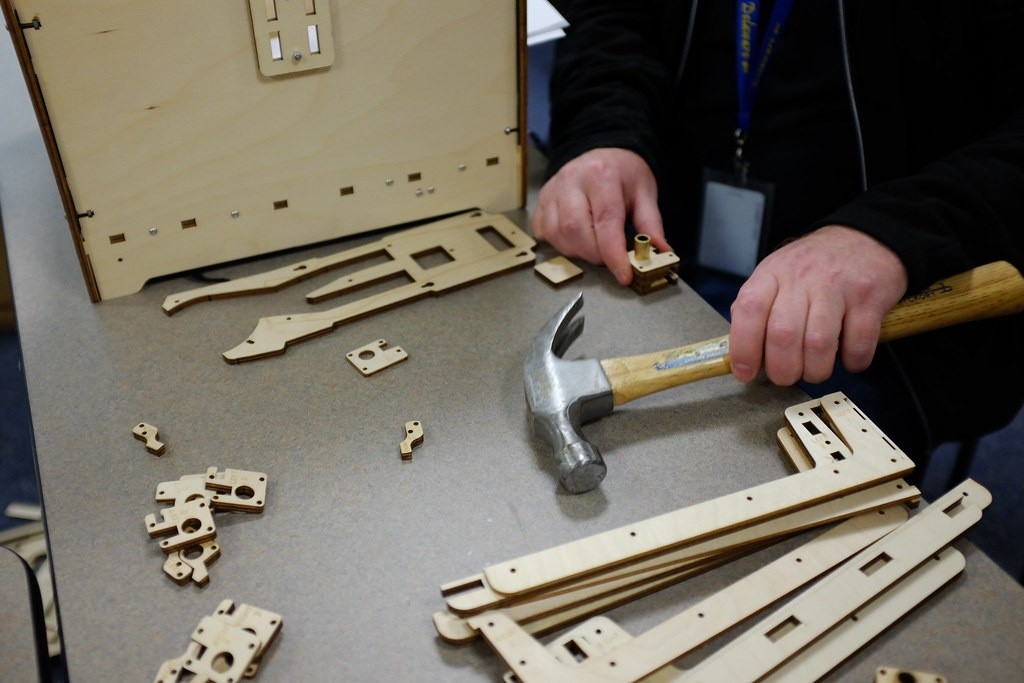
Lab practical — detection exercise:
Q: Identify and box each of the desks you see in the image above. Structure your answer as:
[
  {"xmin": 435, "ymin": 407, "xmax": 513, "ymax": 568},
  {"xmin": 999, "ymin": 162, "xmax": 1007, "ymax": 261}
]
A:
[{"xmin": 0, "ymin": 10, "xmax": 1024, "ymax": 683}]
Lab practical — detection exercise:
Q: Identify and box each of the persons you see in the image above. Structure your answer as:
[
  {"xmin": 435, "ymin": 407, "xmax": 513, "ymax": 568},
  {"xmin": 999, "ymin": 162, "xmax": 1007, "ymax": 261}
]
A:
[{"xmin": 532, "ymin": 0, "xmax": 1024, "ymax": 505}]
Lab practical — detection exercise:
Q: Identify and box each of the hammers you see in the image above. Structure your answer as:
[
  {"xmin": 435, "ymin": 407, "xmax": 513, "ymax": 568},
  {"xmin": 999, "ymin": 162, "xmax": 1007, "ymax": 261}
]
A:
[{"xmin": 521, "ymin": 261, "xmax": 1024, "ymax": 494}]
